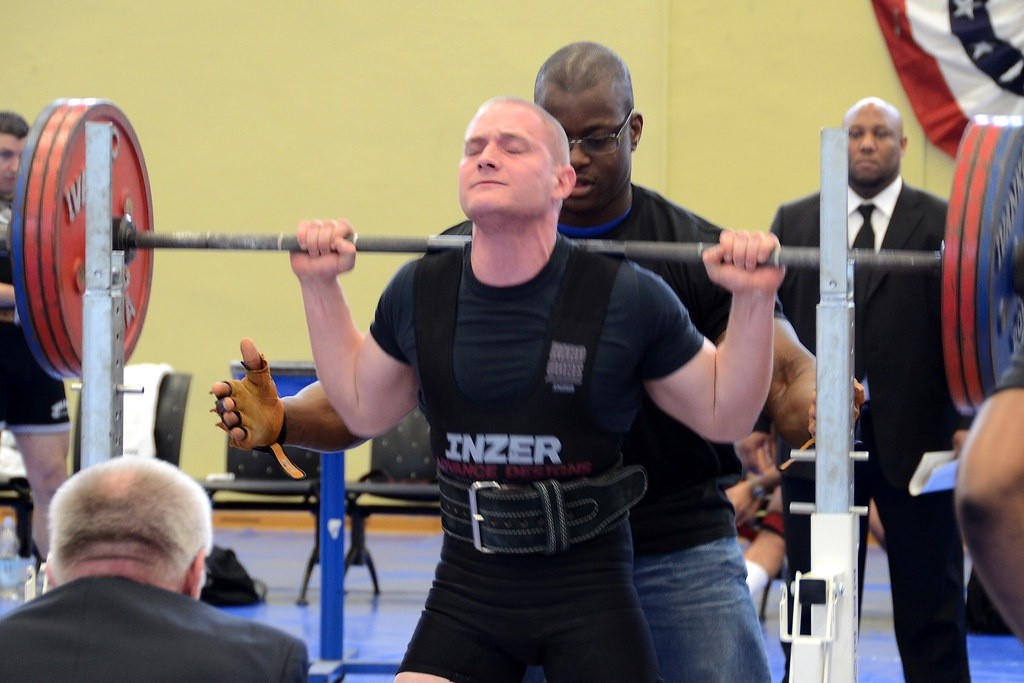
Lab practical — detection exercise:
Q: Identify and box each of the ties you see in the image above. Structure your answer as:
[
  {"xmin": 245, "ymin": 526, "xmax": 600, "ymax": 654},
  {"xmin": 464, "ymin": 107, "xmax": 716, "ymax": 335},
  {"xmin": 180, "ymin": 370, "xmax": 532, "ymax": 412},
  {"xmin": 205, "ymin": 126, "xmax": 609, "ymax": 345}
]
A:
[{"xmin": 849, "ymin": 203, "xmax": 878, "ymax": 302}]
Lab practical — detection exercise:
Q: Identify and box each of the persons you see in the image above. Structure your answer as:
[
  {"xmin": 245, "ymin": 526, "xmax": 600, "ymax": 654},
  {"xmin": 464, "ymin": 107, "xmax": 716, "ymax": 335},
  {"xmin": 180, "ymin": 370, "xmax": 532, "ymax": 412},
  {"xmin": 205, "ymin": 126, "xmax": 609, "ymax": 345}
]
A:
[
  {"xmin": 954, "ymin": 339, "xmax": 1024, "ymax": 642},
  {"xmin": 212, "ymin": 41, "xmax": 865, "ymax": 683},
  {"xmin": 0, "ymin": 110, "xmax": 71, "ymax": 561},
  {"xmin": 290, "ymin": 96, "xmax": 785, "ymax": 683},
  {"xmin": 768, "ymin": 97, "xmax": 971, "ymax": 683},
  {"xmin": 0, "ymin": 454, "xmax": 308, "ymax": 683},
  {"xmin": 722, "ymin": 431, "xmax": 786, "ymax": 618}
]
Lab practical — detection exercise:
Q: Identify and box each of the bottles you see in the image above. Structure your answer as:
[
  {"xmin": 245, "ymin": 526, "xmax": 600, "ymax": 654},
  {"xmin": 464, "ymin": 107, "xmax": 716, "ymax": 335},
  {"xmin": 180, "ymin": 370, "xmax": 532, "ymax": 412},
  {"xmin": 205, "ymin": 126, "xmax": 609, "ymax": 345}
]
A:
[{"xmin": 0, "ymin": 516, "xmax": 20, "ymax": 601}]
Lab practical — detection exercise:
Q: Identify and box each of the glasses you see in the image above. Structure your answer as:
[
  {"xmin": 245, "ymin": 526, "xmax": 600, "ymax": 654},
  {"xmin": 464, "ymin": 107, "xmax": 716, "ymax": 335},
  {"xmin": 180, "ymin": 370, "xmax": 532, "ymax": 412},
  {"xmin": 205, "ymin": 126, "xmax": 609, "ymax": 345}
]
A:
[{"xmin": 569, "ymin": 108, "xmax": 632, "ymax": 156}]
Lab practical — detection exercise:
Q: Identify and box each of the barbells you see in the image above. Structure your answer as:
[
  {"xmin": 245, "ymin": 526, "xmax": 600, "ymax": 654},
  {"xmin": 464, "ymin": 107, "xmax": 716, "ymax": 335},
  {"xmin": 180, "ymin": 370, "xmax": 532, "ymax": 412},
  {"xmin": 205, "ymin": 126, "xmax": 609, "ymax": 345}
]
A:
[{"xmin": 0, "ymin": 96, "xmax": 1024, "ymax": 423}]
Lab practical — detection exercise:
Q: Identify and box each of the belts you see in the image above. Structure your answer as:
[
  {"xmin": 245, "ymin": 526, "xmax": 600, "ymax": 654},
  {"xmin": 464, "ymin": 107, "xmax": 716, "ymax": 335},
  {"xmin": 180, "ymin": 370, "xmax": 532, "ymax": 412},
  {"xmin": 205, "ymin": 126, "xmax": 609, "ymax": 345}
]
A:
[{"xmin": 437, "ymin": 453, "xmax": 646, "ymax": 555}]
